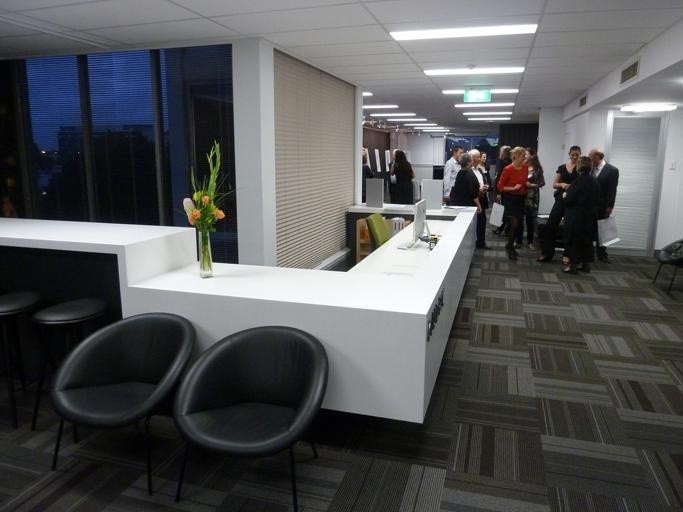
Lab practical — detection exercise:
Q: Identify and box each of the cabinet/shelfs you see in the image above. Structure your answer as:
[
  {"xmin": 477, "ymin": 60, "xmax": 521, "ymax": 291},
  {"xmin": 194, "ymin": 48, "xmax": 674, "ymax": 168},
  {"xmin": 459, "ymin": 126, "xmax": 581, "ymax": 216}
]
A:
[{"xmin": 355, "ymin": 217, "xmax": 411, "ymax": 263}]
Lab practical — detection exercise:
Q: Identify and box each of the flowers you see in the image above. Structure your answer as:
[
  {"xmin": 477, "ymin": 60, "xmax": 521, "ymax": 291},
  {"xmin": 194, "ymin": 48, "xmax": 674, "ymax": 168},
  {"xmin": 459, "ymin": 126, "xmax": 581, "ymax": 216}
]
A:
[{"xmin": 181, "ymin": 139, "xmax": 237, "ymax": 239}]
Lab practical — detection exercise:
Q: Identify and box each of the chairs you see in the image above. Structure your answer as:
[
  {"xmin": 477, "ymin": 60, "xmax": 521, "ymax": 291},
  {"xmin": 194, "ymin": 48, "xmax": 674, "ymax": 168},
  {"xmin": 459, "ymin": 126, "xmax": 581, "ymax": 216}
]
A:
[
  {"xmin": 173, "ymin": 326, "xmax": 329, "ymax": 512},
  {"xmin": 50, "ymin": 312, "xmax": 195, "ymax": 496},
  {"xmin": 653, "ymin": 240, "xmax": 683, "ymax": 293},
  {"xmin": 366, "ymin": 214, "xmax": 389, "ymax": 251}
]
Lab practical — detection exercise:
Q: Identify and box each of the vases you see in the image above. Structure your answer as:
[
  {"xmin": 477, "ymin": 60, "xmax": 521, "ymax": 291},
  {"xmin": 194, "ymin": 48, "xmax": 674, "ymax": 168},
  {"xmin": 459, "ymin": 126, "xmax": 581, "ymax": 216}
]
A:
[{"xmin": 193, "ymin": 232, "xmax": 221, "ymax": 280}]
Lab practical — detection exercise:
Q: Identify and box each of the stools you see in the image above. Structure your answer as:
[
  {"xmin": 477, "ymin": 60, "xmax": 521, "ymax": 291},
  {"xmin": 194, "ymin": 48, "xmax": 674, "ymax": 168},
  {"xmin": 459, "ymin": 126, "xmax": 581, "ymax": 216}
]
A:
[{"xmin": 0, "ymin": 289, "xmax": 111, "ymax": 431}]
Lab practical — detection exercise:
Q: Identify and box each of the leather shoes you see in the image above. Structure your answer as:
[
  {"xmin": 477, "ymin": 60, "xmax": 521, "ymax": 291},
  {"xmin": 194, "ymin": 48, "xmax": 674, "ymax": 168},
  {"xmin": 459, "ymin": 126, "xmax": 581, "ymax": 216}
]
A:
[
  {"xmin": 561, "ymin": 256, "xmax": 590, "ymax": 274},
  {"xmin": 505, "ymin": 243, "xmax": 518, "ymax": 261},
  {"xmin": 598, "ymin": 251, "xmax": 611, "ymax": 262}
]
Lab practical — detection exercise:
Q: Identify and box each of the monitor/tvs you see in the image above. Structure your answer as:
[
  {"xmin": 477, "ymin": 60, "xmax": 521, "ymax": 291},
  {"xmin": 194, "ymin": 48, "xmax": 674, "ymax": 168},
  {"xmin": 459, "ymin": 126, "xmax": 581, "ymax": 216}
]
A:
[{"xmin": 414, "ymin": 199, "xmax": 431, "ymax": 245}]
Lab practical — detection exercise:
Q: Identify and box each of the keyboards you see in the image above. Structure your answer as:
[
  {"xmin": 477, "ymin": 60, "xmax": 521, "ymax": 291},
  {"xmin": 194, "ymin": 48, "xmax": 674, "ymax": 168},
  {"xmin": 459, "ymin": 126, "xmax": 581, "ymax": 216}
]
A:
[{"xmin": 396, "ymin": 239, "xmax": 414, "ymax": 250}]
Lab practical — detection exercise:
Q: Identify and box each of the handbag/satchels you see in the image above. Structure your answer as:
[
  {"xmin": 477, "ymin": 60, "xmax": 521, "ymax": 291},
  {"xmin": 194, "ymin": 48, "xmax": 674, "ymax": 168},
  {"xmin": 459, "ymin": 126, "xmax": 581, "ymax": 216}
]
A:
[
  {"xmin": 489, "ymin": 201, "xmax": 504, "ymax": 228},
  {"xmin": 598, "ymin": 215, "xmax": 621, "ymax": 248}
]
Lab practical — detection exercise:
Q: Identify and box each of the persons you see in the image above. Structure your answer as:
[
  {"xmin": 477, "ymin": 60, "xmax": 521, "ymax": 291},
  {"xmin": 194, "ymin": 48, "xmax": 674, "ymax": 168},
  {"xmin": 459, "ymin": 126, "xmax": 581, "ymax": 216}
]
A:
[
  {"xmin": 443, "ymin": 145, "xmax": 545, "ymax": 260},
  {"xmin": 589, "ymin": 148, "xmax": 619, "ymax": 263},
  {"xmin": 389, "ymin": 150, "xmax": 397, "ymax": 203},
  {"xmin": 362, "ymin": 153, "xmax": 374, "ymax": 202},
  {"xmin": 536, "ymin": 146, "xmax": 581, "ymax": 265},
  {"xmin": 561, "ymin": 156, "xmax": 599, "ymax": 273},
  {"xmin": 390, "ymin": 150, "xmax": 415, "ymax": 204}
]
